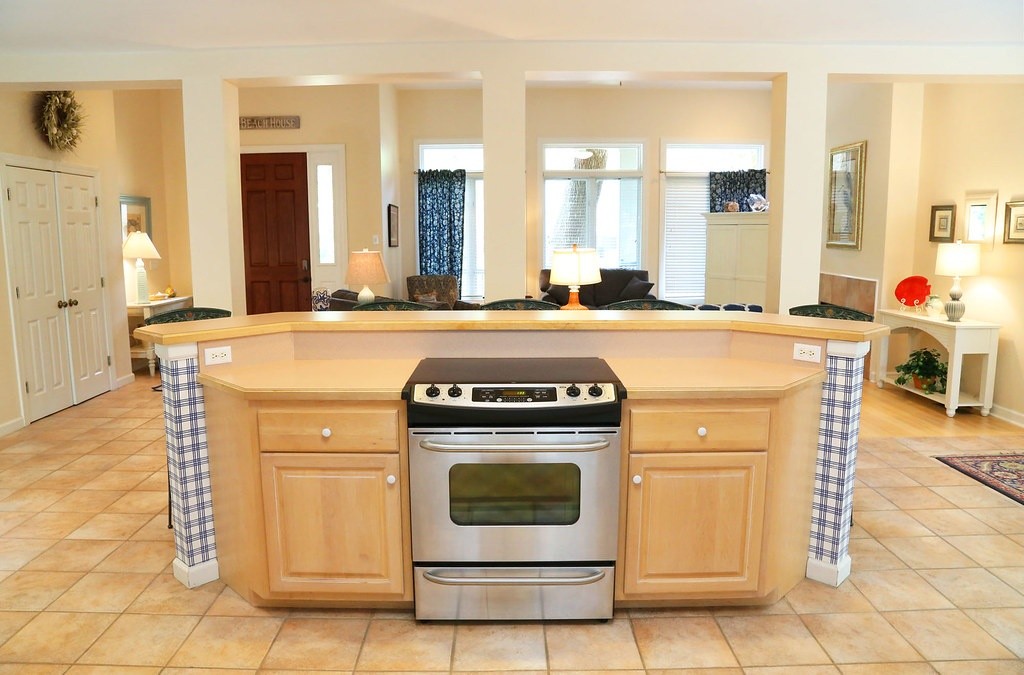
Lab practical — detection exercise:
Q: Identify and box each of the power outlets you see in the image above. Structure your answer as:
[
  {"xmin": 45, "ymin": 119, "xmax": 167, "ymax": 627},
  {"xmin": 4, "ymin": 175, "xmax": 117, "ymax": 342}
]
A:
[
  {"xmin": 793, "ymin": 342, "xmax": 822, "ymax": 364},
  {"xmin": 205, "ymin": 346, "xmax": 234, "ymax": 366}
]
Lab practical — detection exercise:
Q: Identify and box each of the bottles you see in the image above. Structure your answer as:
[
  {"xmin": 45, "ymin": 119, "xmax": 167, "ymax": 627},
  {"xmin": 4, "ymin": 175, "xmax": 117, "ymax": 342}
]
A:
[{"xmin": 925, "ymin": 294, "xmax": 943, "ymax": 320}]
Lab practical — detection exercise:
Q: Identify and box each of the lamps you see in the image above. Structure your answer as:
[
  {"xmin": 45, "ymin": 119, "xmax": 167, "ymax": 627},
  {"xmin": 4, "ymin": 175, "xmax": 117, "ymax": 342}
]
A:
[
  {"xmin": 547, "ymin": 244, "xmax": 603, "ymax": 307},
  {"xmin": 345, "ymin": 249, "xmax": 391, "ymax": 304},
  {"xmin": 122, "ymin": 232, "xmax": 162, "ymax": 304},
  {"xmin": 935, "ymin": 243, "xmax": 981, "ymax": 322}
]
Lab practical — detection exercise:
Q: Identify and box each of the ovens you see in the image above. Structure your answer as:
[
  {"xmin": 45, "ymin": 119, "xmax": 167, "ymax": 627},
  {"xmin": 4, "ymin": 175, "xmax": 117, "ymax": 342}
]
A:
[{"xmin": 408, "ymin": 383, "xmax": 622, "ymax": 625}]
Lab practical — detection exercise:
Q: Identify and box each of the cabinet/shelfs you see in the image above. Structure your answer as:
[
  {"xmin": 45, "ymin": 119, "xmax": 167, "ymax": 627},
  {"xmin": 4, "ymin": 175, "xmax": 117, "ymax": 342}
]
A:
[
  {"xmin": 625, "ymin": 384, "xmax": 826, "ymax": 605},
  {"xmin": 201, "ymin": 386, "xmax": 410, "ymax": 605}
]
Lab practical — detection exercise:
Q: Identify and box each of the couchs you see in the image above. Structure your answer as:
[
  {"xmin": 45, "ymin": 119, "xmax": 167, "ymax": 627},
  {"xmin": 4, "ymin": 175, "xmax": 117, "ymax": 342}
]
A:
[
  {"xmin": 329, "ymin": 289, "xmax": 558, "ymax": 311},
  {"xmin": 540, "ymin": 269, "xmax": 656, "ymax": 308},
  {"xmin": 407, "ymin": 273, "xmax": 481, "ymax": 310}
]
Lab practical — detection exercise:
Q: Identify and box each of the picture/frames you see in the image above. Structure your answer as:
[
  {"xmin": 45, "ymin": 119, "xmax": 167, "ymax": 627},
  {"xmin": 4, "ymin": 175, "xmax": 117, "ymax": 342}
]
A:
[
  {"xmin": 119, "ymin": 196, "xmax": 153, "ymax": 242},
  {"xmin": 962, "ymin": 193, "xmax": 997, "ymax": 252},
  {"xmin": 1002, "ymin": 201, "xmax": 1024, "ymax": 244},
  {"xmin": 828, "ymin": 139, "xmax": 868, "ymax": 250},
  {"xmin": 928, "ymin": 204, "xmax": 956, "ymax": 242},
  {"xmin": 389, "ymin": 204, "xmax": 399, "ymax": 247}
]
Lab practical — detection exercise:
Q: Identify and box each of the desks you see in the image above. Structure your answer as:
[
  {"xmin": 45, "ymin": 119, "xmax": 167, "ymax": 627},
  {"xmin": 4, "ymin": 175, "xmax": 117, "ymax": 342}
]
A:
[
  {"xmin": 126, "ymin": 296, "xmax": 193, "ymax": 377},
  {"xmin": 876, "ymin": 310, "xmax": 1001, "ymax": 417}
]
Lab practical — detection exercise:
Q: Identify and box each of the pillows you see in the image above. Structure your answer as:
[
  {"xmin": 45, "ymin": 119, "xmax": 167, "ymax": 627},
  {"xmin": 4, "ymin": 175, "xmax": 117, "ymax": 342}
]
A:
[
  {"xmin": 596, "ymin": 270, "xmax": 623, "ymax": 306},
  {"xmin": 578, "ymin": 284, "xmax": 595, "ymax": 306},
  {"xmin": 617, "ymin": 276, "xmax": 655, "ymax": 300},
  {"xmin": 415, "ymin": 291, "xmax": 438, "ymax": 303},
  {"xmin": 545, "ymin": 285, "xmax": 569, "ymax": 305}
]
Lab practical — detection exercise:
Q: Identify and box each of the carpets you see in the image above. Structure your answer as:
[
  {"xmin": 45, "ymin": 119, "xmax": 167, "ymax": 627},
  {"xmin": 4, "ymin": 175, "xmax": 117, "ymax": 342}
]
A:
[{"xmin": 931, "ymin": 450, "xmax": 1024, "ymax": 506}]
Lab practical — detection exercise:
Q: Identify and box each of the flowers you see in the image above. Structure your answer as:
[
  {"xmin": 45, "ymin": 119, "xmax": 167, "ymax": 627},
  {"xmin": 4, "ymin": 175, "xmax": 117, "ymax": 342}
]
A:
[{"xmin": 33, "ymin": 90, "xmax": 94, "ymax": 153}]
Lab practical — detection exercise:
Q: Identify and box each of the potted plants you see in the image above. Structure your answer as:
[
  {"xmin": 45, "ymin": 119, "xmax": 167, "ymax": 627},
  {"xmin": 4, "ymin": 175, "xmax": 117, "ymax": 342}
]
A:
[{"xmin": 895, "ymin": 347, "xmax": 947, "ymax": 394}]
